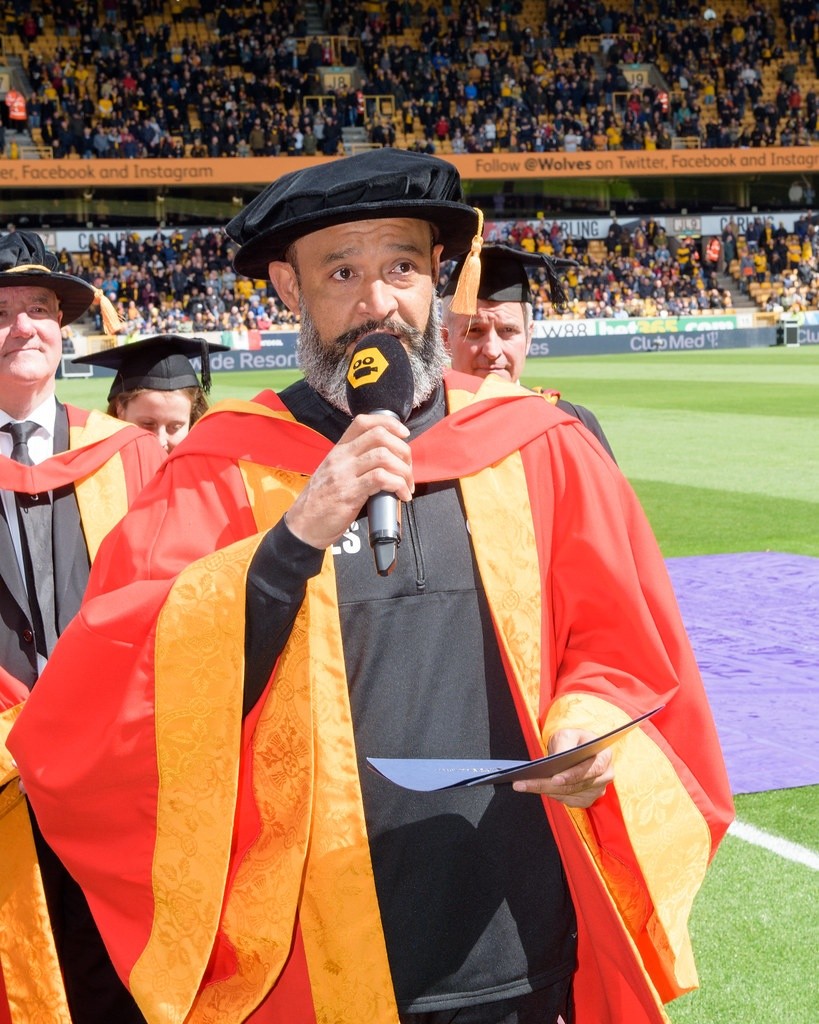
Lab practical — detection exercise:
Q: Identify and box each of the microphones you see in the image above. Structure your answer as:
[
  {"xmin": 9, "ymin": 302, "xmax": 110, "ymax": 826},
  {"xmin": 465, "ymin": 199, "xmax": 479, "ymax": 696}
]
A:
[{"xmin": 346, "ymin": 332, "xmax": 415, "ymax": 576}]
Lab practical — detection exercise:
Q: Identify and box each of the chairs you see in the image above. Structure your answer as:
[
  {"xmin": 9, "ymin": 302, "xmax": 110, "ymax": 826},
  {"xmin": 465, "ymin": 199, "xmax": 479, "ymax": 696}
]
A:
[{"xmin": 0, "ymin": 0, "xmax": 818, "ymax": 335}]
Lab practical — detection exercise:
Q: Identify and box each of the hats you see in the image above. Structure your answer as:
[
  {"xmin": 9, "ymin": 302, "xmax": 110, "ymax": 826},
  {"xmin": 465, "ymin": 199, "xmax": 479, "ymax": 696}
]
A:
[
  {"xmin": 1, "ymin": 229, "xmax": 120, "ymax": 338},
  {"xmin": 438, "ymin": 239, "xmax": 582, "ymax": 315},
  {"xmin": 214, "ymin": 149, "xmax": 494, "ymax": 319},
  {"xmin": 69, "ymin": 333, "xmax": 234, "ymax": 394}
]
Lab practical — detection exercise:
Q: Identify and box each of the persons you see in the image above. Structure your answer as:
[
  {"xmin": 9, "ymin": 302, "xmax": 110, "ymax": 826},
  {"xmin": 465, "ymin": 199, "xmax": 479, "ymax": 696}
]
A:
[
  {"xmin": 432, "ymin": 237, "xmax": 624, "ymax": 468},
  {"xmin": 66, "ymin": 330, "xmax": 230, "ymax": 460},
  {"xmin": 8, "ymin": 142, "xmax": 737, "ymax": 1024},
  {"xmin": 0, "ymin": 0, "xmax": 817, "ymax": 361},
  {"xmin": 0, "ymin": 228, "xmax": 176, "ymax": 1017}
]
